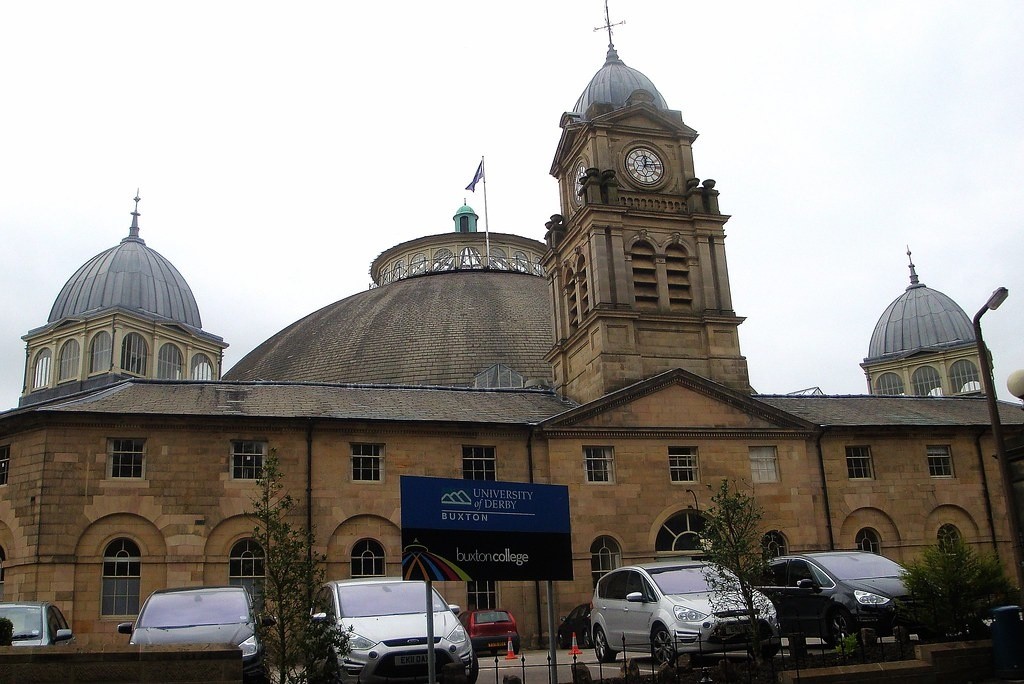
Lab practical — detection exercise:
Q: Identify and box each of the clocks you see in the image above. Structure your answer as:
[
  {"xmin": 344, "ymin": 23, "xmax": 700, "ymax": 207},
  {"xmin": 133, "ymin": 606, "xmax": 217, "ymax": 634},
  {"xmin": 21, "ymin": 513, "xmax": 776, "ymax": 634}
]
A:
[
  {"xmin": 573, "ymin": 164, "xmax": 587, "ymax": 203},
  {"xmin": 625, "ymin": 148, "xmax": 664, "ymax": 185}
]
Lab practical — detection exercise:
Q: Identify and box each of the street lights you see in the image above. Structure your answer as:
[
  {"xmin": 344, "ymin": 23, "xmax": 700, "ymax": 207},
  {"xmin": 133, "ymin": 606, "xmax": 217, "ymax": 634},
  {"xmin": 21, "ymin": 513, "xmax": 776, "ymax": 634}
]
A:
[{"xmin": 972, "ymin": 285, "xmax": 1024, "ymax": 615}]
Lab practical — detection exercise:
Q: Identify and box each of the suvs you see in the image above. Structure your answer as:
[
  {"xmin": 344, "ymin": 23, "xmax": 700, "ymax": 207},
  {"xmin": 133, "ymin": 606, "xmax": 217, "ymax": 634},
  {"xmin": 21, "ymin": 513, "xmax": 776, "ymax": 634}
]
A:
[{"xmin": 457, "ymin": 607, "xmax": 521, "ymax": 655}]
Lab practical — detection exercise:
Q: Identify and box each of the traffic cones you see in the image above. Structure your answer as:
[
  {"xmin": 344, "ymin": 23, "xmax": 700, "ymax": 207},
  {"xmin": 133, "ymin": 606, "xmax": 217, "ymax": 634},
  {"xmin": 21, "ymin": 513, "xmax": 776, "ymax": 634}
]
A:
[
  {"xmin": 504, "ymin": 635, "xmax": 519, "ymax": 660},
  {"xmin": 568, "ymin": 631, "xmax": 583, "ymax": 655}
]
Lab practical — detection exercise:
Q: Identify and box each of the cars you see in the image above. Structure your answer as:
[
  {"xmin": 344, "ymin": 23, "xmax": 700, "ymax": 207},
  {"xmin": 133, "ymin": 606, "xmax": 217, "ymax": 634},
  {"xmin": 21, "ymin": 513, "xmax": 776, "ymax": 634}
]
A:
[
  {"xmin": 0, "ymin": 599, "xmax": 77, "ymax": 646},
  {"xmin": 587, "ymin": 560, "xmax": 783, "ymax": 670},
  {"xmin": 556, "ymin": 602, "xmax": 596, "ymax": 649},
  {"xmin": 742, "ymin": 547, "xmax": 945, "ymax": 650},
  {"xmin": 116, "ymin": 585, "xmax": 277, "ymax": 684},
  {"xmin": 304, "ymin": 575, "xmax": 480, "ymax": 684}
]
